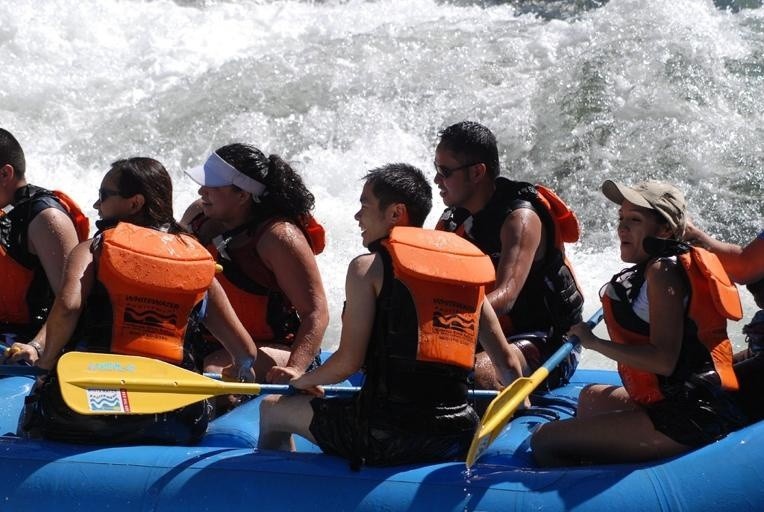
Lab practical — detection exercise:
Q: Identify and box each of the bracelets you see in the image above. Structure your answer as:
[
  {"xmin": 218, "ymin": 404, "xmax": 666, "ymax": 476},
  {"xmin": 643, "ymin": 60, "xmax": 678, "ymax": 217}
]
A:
[{"xmin": 24, "ymin": 341, "xmax": 45, "ymax": 366}]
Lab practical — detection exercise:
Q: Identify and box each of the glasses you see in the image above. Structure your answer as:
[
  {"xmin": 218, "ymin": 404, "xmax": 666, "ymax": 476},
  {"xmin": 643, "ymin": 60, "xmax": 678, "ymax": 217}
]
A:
[
  {"xmin": 433, "ymin": 161, "xmax": 489, "ymax": 179},
  {"xmin": 99, "ymin": 187, "xmax": 139, "ymax": 202}
]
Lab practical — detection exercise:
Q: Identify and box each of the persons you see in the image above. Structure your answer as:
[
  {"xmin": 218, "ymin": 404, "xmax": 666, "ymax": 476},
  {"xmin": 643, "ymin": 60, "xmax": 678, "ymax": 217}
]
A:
[
  {"xmin": 178, "ymin": 144, "xmax": 329, "ymax": 417},
  {"xmin": 256, "ymin": 163, "xmax": 531, "ymax": 472},
  {"xmin": 686, "ymin": 224, "xmax": 764, "ymax": 411},
  {"xmin": 18, "ymin": 157, "xmax": 257, "ymax": 449},
  {"xmin": 435, "ymin": 122, "xmax": 584, "ymax": 390},
  {"xmin": 530, "ymin": 179, "xmax": 742, "ymax": 469},
  {"xmin": 0, "ymin": 128, "xmax": 80, "ymax": 367}
]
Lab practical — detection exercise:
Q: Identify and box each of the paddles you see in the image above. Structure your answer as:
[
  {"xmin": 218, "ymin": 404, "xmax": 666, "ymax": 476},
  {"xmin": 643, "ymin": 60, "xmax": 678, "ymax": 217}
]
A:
[
  {"xmin": 56, "ymin": 350, "xmax": 502, "ymax": 417},
  {"xmin": 461, "ymin": 305, "xmax": 604, "ymax": 471}
]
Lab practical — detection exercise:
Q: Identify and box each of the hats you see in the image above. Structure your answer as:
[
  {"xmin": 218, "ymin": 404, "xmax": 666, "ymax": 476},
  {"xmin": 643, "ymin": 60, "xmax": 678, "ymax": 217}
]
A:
[
  {"xmin": 182, "ymin": 151, "xmax": 270, "ymax": 204},
  {"xmin": 601, "ymin": 177, "xmax": 687, "ymax": 242}
]
